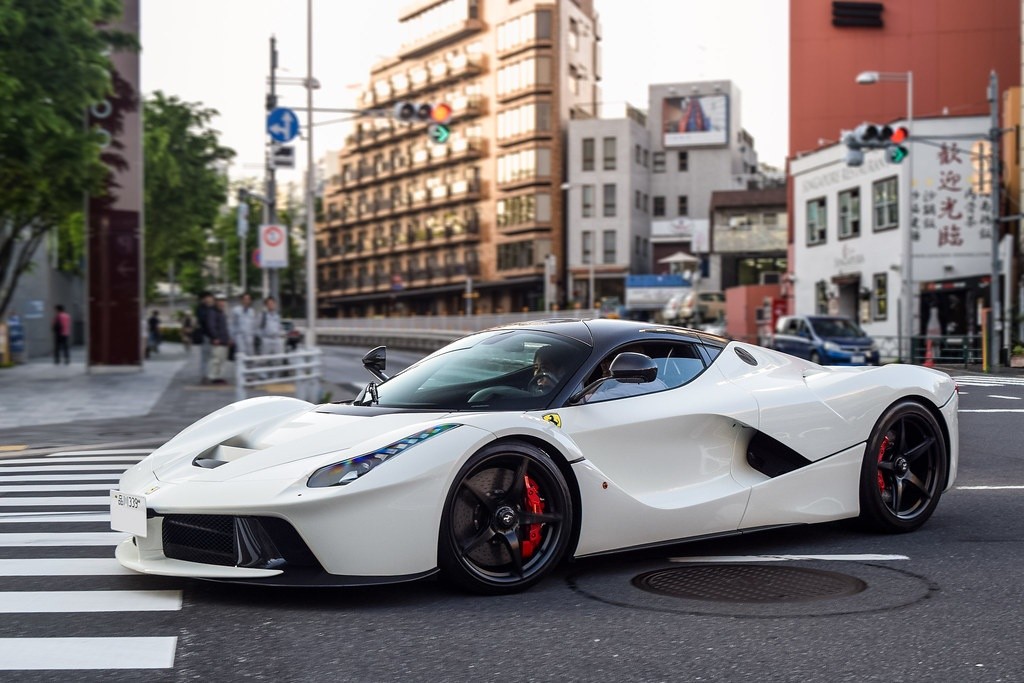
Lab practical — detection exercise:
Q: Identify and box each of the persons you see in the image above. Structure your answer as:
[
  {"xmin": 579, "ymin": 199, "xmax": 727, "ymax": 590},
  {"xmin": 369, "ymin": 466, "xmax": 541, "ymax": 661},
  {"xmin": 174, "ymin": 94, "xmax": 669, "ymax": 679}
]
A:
[
  {"xmin": 255, "ymin": 295, "xmax": 286, "ymax": 381},
  {"xmin": 533, "ymin": 345, "xmax": 568, "ymax": 398},
  {"xmin": 194, "ymin": 291, "xmax": 216, "ymax": 382},
  {"xmin": 226, "ymin": 293, "xmax": 256, "ymax": 357},
  {"xmin": 50, "ymin": 304, "xmax": 72, "ymax": 366},
  {"xmin": 201, "ymin": 294, "xmax": 230, "ymax": 383},
  {"xmin": 146, "ymin": 310, "xmax": 161, "ymax": 354},
  {"xmin": 584, "ymin": 343, "xmax": 668, "ymax": 402}
]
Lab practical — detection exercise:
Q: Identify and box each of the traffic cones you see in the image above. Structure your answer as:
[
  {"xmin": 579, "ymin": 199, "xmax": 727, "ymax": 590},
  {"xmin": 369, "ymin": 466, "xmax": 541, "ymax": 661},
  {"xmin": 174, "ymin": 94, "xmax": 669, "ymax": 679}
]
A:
[{"xmin": 921, "ymin": 339, "xmax": 934, "ymax": 366}]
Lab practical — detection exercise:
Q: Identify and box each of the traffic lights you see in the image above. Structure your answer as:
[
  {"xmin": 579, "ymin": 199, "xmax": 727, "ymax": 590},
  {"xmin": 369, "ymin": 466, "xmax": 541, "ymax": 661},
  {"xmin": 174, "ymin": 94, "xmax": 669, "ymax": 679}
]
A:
[
  {"xmin": 842, "ymin": 123, "xmax": 909, "ymax": 165},
  {"xmin": 394, "ymin": 99, "xmax": 454, "ymax": 144}
]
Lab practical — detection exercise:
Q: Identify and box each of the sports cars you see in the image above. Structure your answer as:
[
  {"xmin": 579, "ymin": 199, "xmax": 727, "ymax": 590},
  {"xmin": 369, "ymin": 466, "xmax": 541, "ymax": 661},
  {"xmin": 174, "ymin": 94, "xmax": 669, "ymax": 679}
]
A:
[{"xmin": 110, "ymin": 317, "xmax": 960, "ymax": 594}]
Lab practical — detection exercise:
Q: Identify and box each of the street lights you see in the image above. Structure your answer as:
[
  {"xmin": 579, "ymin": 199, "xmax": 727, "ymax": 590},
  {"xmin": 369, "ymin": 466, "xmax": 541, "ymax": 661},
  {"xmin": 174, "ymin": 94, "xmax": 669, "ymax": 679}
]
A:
[
  {"xmin": 855, "ymin": 70, "xmax": 914, "ymax": 364},
  {"xmin": 262, "ymin": 75, "xmax": 322, "ymax": 312}
]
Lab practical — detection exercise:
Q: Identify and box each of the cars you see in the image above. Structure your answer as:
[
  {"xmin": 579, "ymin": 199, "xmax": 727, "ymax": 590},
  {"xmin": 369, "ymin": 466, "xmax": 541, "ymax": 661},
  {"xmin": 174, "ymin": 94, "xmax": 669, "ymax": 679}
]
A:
[
  {"xmin": 254, "ymin": 315, "xmax": 304, "ymax": 354},
  {"xmin": 661, "ymin": 290, "xmax": 726, "ymax": 325},
  {"xmin": 770, "ymin": 316, "xmax": 881, "ymax": 365}
]
